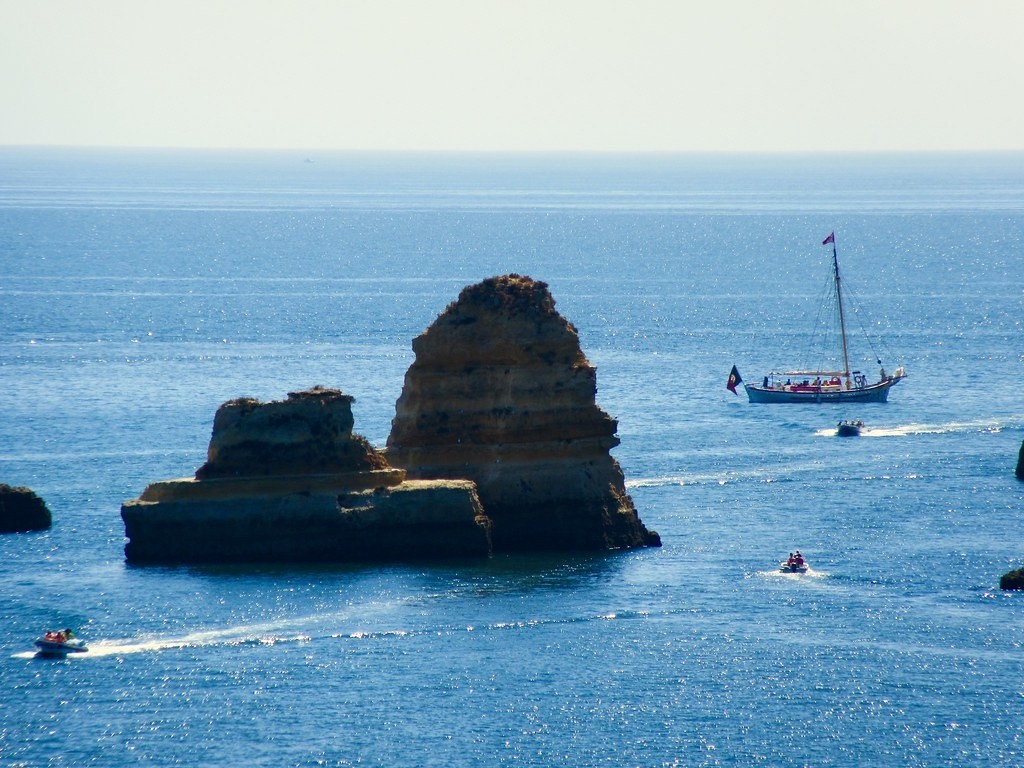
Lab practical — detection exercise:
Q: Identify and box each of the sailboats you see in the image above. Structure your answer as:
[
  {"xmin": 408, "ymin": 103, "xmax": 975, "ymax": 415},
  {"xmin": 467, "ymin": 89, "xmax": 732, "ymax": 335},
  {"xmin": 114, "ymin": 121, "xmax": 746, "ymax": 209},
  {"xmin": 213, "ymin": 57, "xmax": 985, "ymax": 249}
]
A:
[{"xmin": 741, "ymin": 232, "xmax": 908, "ymax": 403}]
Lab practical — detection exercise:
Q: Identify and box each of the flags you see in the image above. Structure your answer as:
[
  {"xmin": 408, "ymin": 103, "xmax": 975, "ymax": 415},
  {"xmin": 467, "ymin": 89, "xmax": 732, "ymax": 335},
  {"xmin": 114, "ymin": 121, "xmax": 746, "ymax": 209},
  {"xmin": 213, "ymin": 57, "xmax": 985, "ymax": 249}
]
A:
[{"xmin": 727, "ymin": 366, "xmax": 742, "ymax": 396}]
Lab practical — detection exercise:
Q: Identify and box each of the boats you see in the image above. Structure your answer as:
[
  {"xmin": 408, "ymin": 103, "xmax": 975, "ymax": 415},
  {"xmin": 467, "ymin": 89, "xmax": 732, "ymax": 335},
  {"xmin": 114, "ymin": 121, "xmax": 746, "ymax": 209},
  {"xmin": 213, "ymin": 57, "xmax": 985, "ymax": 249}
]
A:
[
  {"xmin": 33, "ymin": 636, "xmax": 88, "ymax": 654},
  {"xmin": 779, "ymin": 559, "xmax": 808, "ymax": 573},
  {"xmin": 838, "ymin": 423, "xmax": 860, "ymax": 435}
]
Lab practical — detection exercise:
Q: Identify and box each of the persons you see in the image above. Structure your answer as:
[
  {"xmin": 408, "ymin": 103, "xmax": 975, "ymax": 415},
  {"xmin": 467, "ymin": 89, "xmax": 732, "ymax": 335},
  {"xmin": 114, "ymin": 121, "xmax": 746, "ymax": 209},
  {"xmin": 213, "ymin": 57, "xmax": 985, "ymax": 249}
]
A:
[
  {"xmin": 787, "ymin": 379, "xmax": 791, "ymax": 385},
  {"xmin": 787, "ymin": 550, "xmax": 803, "ymax": 567},
  {"xmin": 776, "ymin": 380, "xmax": 781, "ymax": 387},
  {"xmin": 45, "ymin": 631, "xmax": 65, "ymax": 643},
  {"xmin": 846, "ymin": 420, "xmax": 862, "ymax": 426},
  {"xmin": 817, "ymin": 377, "xmax": 822, "ymax": 391}
]
